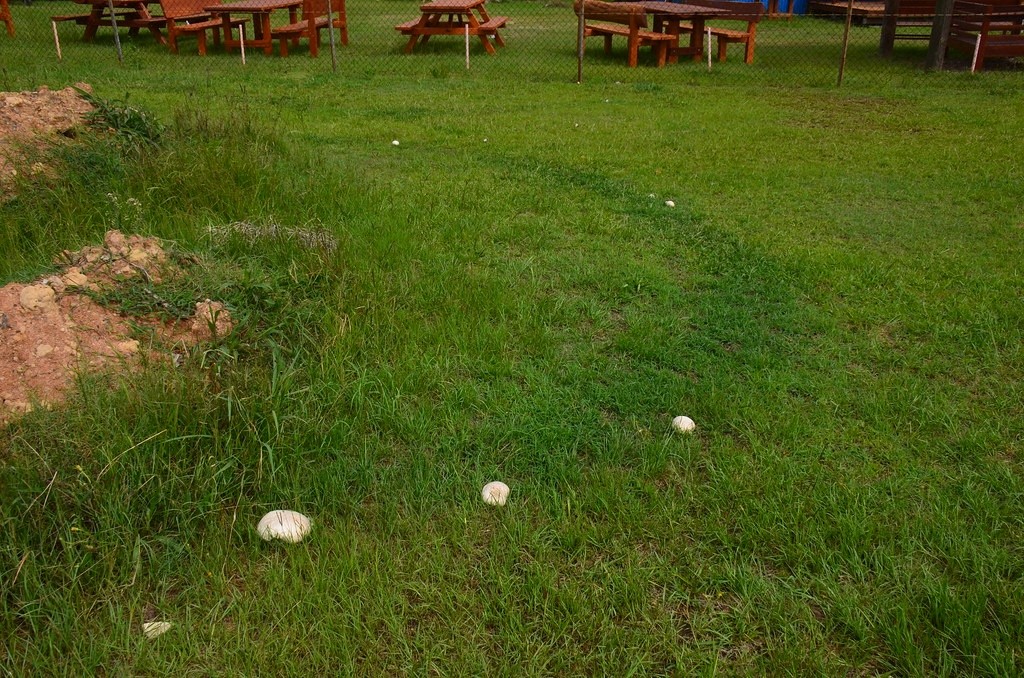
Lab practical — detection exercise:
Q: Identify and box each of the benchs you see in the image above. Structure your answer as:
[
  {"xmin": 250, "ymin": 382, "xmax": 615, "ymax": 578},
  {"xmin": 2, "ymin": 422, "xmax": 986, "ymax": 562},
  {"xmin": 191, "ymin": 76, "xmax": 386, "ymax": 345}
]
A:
[
  {"xmin": 132, "ymin": 13, "xmax": 222, "ymax": 25},
  {"xmin": 478, "ymin": 16, "xmax": 509, "ymax": 30},
  {"xmin": 159, "ymin": 0, "xmax": 250, "ymax": 56},
  {"xmin": 50, "ymin": 11, "xmax": 152, "ymax": 21},
  {"xmin": 395, "ymin": 16, "xmax": 434, "ymax": 30},
  {"xmin": 272, "ymin": 0, "xmax": 349, "ymax": 58},
  {"xmin": 663, "ymin": 0, "xmax": 764, "ymax": 64},
  {"xmin": 573, "ymin": 0, "xmax": 676, "ymax": 67}
]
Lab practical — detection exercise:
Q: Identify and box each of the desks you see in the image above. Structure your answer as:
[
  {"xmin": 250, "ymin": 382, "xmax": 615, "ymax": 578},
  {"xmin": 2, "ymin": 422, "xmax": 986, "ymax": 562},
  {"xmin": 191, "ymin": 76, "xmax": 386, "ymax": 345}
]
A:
[
  {"xmin": 395, "ymin": 0, "xmax": 509, "ymax": 55},
  {"xmin": 203, "ymin": 0, "xmax": 304, "ymax": 56},
  {"xmin": 610, "ymin": 1, "xmax": 731, "ymax": 63},
  {"xmin": 73, "ymin": 0, "xmax": 210, "ymax": 46}
]
